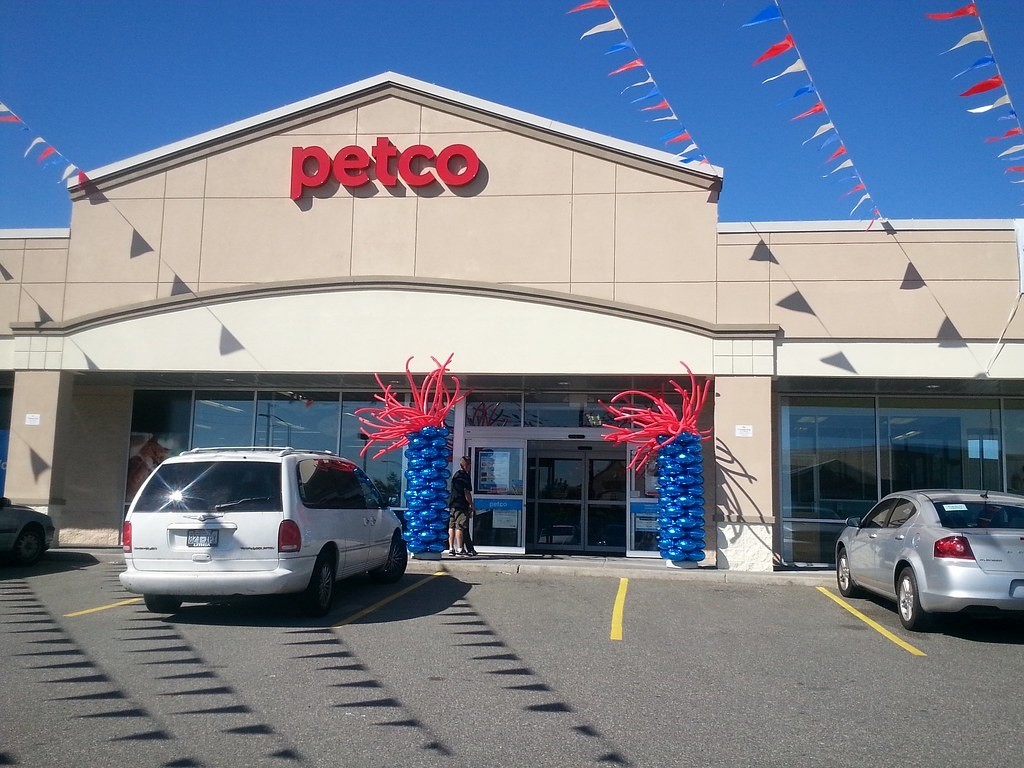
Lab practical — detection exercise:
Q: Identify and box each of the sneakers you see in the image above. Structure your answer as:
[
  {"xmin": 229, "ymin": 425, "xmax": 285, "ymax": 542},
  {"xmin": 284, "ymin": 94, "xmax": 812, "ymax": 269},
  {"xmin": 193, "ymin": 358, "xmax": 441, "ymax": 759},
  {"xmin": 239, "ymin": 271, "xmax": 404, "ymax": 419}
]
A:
[
  {"xmin": 449, "ymin": 549, "xmax": 455, "ymax": 556},
  {"xmin": 455, "ymin": 549, "xmax": 472, "ymax": 557}
]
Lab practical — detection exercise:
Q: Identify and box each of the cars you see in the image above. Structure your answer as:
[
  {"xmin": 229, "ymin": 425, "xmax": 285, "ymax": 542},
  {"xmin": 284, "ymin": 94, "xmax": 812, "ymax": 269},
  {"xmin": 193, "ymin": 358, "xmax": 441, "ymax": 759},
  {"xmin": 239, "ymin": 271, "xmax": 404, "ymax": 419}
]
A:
[
  {"xmin": 834, "ymin": 489, "xmax": 1024, "ymax": 630},
  {"xmin": 0, "ymin": 497, "xmax": 55, "ymax": 561}
]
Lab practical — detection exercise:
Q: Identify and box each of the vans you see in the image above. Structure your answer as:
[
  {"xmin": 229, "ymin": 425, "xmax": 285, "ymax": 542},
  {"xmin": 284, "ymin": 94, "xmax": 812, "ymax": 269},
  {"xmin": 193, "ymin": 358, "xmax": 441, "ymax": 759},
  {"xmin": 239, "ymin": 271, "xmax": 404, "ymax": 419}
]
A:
[{"xmin": 784, "ymin": 505, "xmax": 841, "ymax": 527}]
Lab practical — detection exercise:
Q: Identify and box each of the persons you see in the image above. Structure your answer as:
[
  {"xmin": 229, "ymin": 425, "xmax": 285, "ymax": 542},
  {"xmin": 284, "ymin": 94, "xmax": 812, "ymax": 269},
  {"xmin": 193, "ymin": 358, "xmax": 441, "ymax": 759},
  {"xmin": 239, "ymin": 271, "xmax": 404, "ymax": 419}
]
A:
[{"xmin": 448, "ymin": 456, "xmax": 475, "ymax": 557}]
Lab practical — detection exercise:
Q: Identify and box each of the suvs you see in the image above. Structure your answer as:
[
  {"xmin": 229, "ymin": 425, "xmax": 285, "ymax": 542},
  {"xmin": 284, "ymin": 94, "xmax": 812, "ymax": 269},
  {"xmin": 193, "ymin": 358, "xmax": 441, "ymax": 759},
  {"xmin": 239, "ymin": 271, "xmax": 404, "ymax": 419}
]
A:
[{"xmin": 122, "ymin": 444, "xmax": 410, "ymax": 613}]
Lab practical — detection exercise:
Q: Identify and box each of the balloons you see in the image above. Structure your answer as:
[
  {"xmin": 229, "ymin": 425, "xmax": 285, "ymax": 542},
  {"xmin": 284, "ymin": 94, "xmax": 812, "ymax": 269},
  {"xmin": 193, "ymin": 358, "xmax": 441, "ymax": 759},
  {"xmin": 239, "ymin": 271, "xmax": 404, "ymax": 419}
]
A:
[
  {"xmin": 355, "ymin": 352, "xmax": 476, "ymax": 554},
  {"xmin": 597, "ymin": 360, "xmax": 713, "ymax": 563}
]
